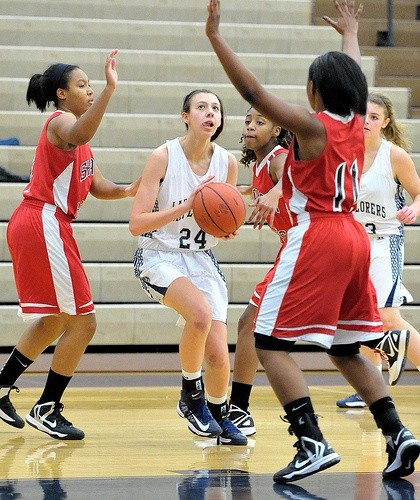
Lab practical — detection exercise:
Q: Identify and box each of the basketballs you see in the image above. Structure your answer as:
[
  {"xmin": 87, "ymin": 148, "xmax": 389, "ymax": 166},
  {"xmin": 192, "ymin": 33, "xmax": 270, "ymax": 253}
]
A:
[{"xmin": 193, "ymin": 183, "xmax": 246, "ymax": 237}]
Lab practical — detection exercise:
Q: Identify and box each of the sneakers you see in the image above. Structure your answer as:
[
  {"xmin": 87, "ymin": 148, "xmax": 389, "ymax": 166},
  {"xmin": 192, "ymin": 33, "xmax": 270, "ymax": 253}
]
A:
[
  {"xmin": 373, "ymin": 329, "xmax": 410, "ymax": 387},
  {"xmin": 272, "ymin": 434, "xmax": 341, "ymax": 483},
  {"xmin": 218, "ymin": 412, "xmax": 248, "ymax": 445},
  {"xmin": 381, "ymin": 422, "xmax": 420, "ymax": 479},
  {"xmin": 25, "ymin": 401, "xmax": 85, "ymax": 440},
  {"xmin": 0, "ymin": 385, "xmax": 25, "ymax": 429},
  {"xmin": 337, "ymin": 392, "xmax": 368, "ymax": 407},
  {"xmin": 227, "ymin": 400, "xmax": 256, "ymax": 436},
  {"xmin": 176, "ymin": 388, "xmax": 223, "ymax": 437}
]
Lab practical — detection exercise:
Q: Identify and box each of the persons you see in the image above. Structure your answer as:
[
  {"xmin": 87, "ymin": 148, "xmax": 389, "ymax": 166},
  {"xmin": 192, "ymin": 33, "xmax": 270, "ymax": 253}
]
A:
[
  {"xmin": 206, "ymin": 0, "xmax": 420, "ymax": 482},
  {"xmin": 336, "ymin": 94, "xmax": 420, "ymax": 409},
  {"xmin": 229, "ymin": 106, "xmax": 409, "ymax": 436},
  {"xmin": 129, "ymin": 89, "xmax": 249, "ymax": 446},
  {"xmin": 0, "ymin": 49, "xmax": 140, "ymax": 443}
]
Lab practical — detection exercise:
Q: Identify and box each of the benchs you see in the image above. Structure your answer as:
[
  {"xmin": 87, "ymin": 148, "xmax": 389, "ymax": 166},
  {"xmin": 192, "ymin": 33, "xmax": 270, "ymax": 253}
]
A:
[{"xmin": 0, "ymin": 0, "xmax": 420, "ymax": 354}]
